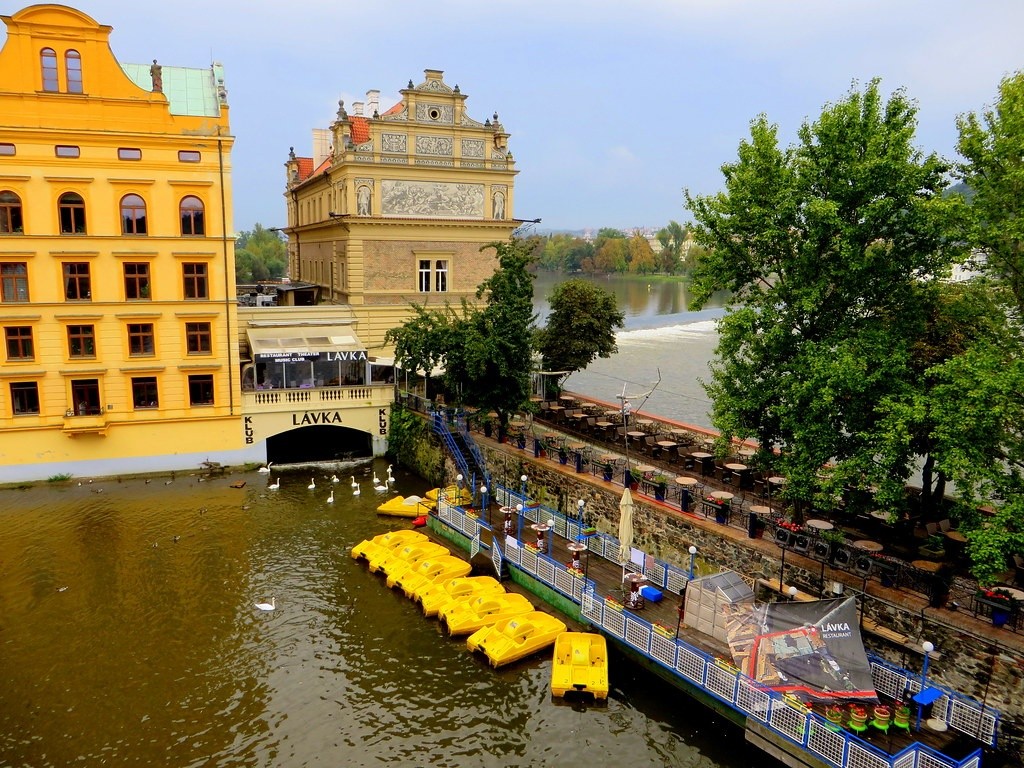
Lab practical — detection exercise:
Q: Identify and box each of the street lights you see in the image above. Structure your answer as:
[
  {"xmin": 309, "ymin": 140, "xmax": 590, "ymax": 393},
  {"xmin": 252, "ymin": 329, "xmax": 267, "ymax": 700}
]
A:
[
  {"xmin": 578, "ymin": 500, "xmax": 585, "ymax": 538},
  {"xmin": 520, "ymin": 474, "xmax": 529, "ymax": 504},
  {"xmin": 689, "ymin": 545, "xmax": 697, "ymax": 580},
  {"xmin": 516, "ymin": 503, "xmax": 525, "ymax": 540},
  {"xmin": 546, "ymin": 518, "xmax": 555, "ymax": 560},
  {"xmin": 479, "ymin": 486, "xmax": 487, "ymax": 521},
  {"xmin": 913, "ymin": 641, "xmax": 934, "ymax": 733},
  {"xmin": 456, "ymin": 473, "xmax": 463, "ymax": 507}
]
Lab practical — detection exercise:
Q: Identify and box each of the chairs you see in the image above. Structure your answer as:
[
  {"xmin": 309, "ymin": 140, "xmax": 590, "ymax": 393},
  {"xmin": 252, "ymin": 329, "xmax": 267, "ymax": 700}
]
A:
[{"xmin": 490, "ymin": 399, "xmax": 1024, "ymax": 619}]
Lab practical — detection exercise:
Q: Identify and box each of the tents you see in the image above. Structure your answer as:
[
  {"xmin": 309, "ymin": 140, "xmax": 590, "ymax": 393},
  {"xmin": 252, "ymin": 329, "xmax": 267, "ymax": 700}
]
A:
[{"xmin": 246, "ymin": 327, "xmax": 368, "ymax": 387}]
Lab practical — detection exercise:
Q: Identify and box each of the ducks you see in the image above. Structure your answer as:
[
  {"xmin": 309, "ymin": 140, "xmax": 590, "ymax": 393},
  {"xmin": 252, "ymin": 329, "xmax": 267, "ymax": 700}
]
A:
[
  {"xmin": 56, "ymin": 586, "xmax": 69, "ymax": 592},
  {"xmin": 77, "ymin": 471, "xmax": 235, "ymax": 495},
  {"xmin": 153, "ymin": 505, "xmax": 252, "ymax": 548}
]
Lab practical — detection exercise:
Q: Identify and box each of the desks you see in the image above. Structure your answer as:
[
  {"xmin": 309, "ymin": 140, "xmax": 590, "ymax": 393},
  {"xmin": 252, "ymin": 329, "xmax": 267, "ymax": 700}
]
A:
[{"xmin": 487, "ymin": 396, "xmax": 1024, "ymax": 603}]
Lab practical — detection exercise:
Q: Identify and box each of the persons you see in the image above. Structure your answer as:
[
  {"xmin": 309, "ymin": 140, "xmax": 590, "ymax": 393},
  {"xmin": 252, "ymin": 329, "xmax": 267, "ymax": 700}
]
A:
[
  {"xmin": 358, "ymin": 188, "xmax": 370, "ymax": 216},
  {"xmin": 258, "ymin": 370, "xmax": 278, "ymax": 390},
  {"xmin": 619, "ymin": 399, "xmax": 633, "ymax": 427},
  {"xmin": 149, "ymin": 60, "xmax": 163, "ymax": 91},
  {"xmin": 493, "ymin": 194, "xmax": 503, "ymax": 219}
]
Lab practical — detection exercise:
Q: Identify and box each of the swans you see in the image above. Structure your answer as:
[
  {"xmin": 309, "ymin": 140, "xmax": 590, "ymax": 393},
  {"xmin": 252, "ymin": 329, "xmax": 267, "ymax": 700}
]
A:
[{"xmin": 258, "ymin": 461, "xmax": 399, "ymax": 504}]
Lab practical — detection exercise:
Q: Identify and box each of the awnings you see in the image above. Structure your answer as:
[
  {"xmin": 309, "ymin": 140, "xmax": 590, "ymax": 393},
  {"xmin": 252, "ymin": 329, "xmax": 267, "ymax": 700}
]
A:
[{"xmin": 531, "ymin": 371, "xmax": 572, "ymax": 391}]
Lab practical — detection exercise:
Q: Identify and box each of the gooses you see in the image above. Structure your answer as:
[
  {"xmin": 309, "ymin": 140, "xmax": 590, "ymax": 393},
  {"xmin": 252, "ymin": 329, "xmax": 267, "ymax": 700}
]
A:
[{"xmin": 254, "ymin": 597, "xmax": 277, "ymax": 613}]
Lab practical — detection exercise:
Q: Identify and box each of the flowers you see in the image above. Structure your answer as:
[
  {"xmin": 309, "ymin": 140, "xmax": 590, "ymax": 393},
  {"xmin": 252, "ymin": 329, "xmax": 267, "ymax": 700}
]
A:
[{"xmin": 443, "ymin": 472, "xmax": 1013, "ymax": 717}]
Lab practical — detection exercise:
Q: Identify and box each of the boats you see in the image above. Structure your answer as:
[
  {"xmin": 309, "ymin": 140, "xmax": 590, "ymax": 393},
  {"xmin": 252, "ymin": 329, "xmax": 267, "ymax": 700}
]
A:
[
  {"xmin": 466, "ymin": 610, "xmax": 568, "ymax": 669},
  {"xmin": 438, "ymin": 593, "xmax": 536, "ymax": 637},
  {"xmin": 376, "ymin": 494, "xmax": 436, "ymax": 518},
  {"xmin": 351, "ymin": 529, "xmax": 430, "ymax": 564},
  {"xmin": 413, "ymin": 575, "xmax": 507, "ymax": 618},
  {"xmin": 384, "ymin": 554, "xmax": 472, "ymax": 600},
  {"xmin": 369, "ymin": 542, "xmax": 450, "ymax": 576},
  {"xmin": 551, "ymin": 632, "xmax": 610, "ymax": 701},
  {"xmin": 423, "ymin": 487, "xmax": 473, "ymax": 506}
]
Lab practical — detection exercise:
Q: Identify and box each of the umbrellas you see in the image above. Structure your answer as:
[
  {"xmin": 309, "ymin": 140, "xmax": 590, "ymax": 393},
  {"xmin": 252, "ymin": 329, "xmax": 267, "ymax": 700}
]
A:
[
  {"xmin": 623, "ymin": 572, "xmax": 648, "ymax": 608},
  {"xmin": 617, "ymin": 488, "xmax": 634, "ymax": 585},
  {"xmin": 499, "ymin": 507, "xmax": 517, "ymax": 535},
  {"xmin": 567, "ymin": 542, "xmax": 588, "ymax": 575},
  {"xmin": 530, "ymin": 523, "xmax": 550, "ymax": 554}
]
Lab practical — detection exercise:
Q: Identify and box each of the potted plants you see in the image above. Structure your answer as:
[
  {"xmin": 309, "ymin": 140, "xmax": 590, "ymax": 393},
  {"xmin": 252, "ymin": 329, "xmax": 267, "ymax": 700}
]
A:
[
  {"xmin": 803, "ymin": 709, "xmax": 908, "ymax": 731},
  {"xmin": 583, "ymin": 528, "xmax": 597, "ymax": 535},
  {"xmin": 476, "ymin": 416, "xmax": 766, "ymax": 539},
  {"xmin": 525, "ymin": 500, "xmax": 541, "ymax": 507}
]
